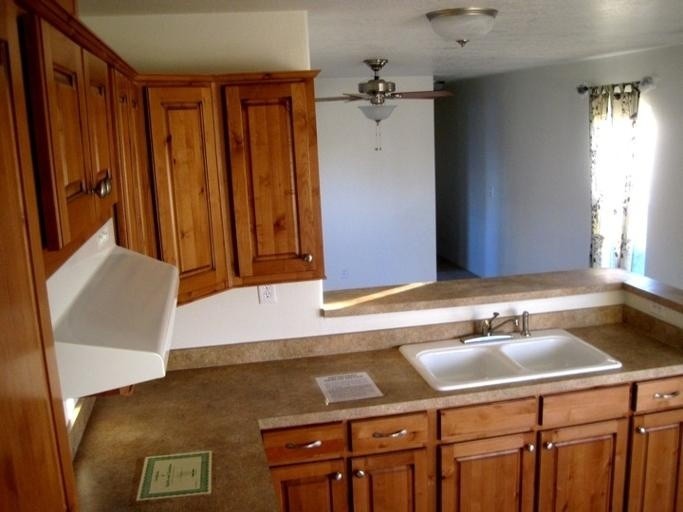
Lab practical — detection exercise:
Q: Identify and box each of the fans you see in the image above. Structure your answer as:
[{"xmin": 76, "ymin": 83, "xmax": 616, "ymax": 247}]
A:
[{"xmin": 312, "ymin": 58, "xmax": 451, "ymax": 107}]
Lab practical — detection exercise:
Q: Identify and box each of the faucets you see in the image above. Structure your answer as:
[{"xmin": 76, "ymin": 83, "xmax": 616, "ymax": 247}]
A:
[{"xmin": 481, "ymin": 313, "xmax": 519, "ymax": 337}]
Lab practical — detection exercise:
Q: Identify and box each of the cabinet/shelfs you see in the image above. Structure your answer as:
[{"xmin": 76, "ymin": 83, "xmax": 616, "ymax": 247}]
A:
[
  {"xmin": 623, "ymin": 376, "xmax": 683, "ymax": 512},
  {"xmin": 129, "ymin": 71, "xmax": 235, "ymax": 307},
  {"xmin": 16, "ymin": 0, "xmax": 115, "ymax": 279},
  {"xmin": 259, "ymin": 409, "xmax": 428, "ymax": 512},
  {"xmin": 435, "ymin": 384, "xmax": 629, "ymax": 512},
  {"xmin": 223, "ymin": 69, "xmax": 327, "ymax": 288},
  {"xmin": 108, "ymin": 47, "xmax": 160, "ymax": 260}
]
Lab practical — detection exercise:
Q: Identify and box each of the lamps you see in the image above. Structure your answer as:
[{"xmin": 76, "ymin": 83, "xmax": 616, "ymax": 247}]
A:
[
  {"xmin": 425, "ymin": 7, "xmax": 498, "ymax": 52},
  {"xmin": 354, "ymin": 101, "xmax": 396, "ymax": 154}
]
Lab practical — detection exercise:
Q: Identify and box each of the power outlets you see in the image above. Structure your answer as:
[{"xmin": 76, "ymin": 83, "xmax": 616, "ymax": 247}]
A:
[{"xmin": 258, "ymin": 283, "xmax": 279, "ymax": 306}]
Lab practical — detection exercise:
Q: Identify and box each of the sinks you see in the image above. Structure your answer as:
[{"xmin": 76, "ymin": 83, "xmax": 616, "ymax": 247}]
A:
[
  {"xmin": 501, "ymin": 327, "xmax": 622, "ymax": 376},
  {"xmin": 399, "ymin": 337, "xmax": 519, "ymax": 392}
]
